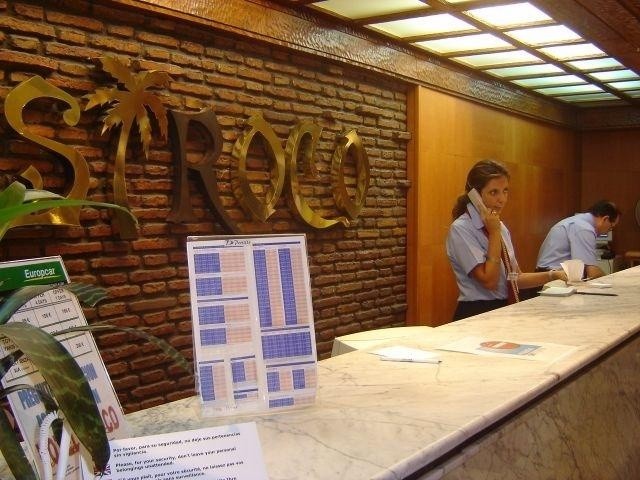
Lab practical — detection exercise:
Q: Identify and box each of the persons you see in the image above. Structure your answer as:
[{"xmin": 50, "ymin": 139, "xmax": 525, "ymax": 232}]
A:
[
  {"xmin": 445, "ymin": 160, "xmax": 567, "ymax": 322},
  {"xmin": 535, "ymin": 200, "xmax": 621, "ymax": 297}
]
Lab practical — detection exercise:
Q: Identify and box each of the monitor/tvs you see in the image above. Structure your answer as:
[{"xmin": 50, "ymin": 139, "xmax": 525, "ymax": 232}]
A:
[{"xmin": 574, "ymin": 212, "xmax": 612, "ymax": 249}]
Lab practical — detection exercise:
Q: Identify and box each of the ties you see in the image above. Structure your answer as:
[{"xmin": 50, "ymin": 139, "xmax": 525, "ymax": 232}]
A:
[{"xmin": 482, "ymin": 225, "xmax": 518, "ymax": 306}]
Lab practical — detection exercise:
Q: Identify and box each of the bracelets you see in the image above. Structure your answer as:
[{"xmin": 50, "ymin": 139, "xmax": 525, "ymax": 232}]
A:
[
  {"xmin": 486, "ymin": 257, "xmax": 500, "ymax": 264},
  {"xmin": 549, "ymin": 269, "xmax": 556, "ymax": 281}
]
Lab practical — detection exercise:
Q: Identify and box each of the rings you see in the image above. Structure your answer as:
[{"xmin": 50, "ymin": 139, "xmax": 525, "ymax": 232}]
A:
[{"xmin": 492, "ymin": 211, "xmax": 496, "ymax": 215}]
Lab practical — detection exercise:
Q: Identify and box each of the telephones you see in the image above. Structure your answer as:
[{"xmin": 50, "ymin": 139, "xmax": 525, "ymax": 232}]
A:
[{"xmin": 467, "ymin": 188, "xmax": 487, "ymax": 214}]
